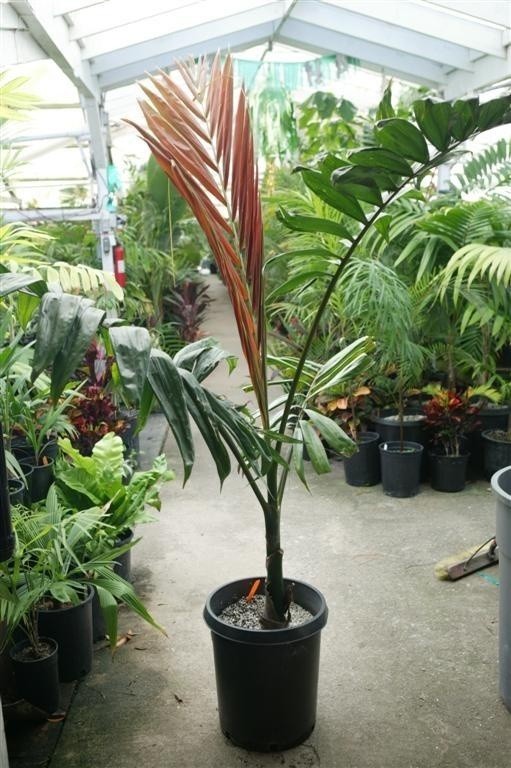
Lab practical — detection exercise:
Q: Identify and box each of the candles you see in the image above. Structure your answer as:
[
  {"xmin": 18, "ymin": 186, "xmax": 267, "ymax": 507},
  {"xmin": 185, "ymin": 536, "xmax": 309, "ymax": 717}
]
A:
[{"xmin": 118, "ymin": 42, "xmax": 510, "ymax": 758}]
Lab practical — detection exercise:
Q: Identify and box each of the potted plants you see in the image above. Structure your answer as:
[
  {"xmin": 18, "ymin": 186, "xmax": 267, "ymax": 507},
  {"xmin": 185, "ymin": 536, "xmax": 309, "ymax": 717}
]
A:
[
  {"xmin": 339, "ymin": 263, "xmax": 506, "ymax": 497},
  {"xmin": 0, "ymin": 305, "xmax": 138, "ymax": 752}
]
